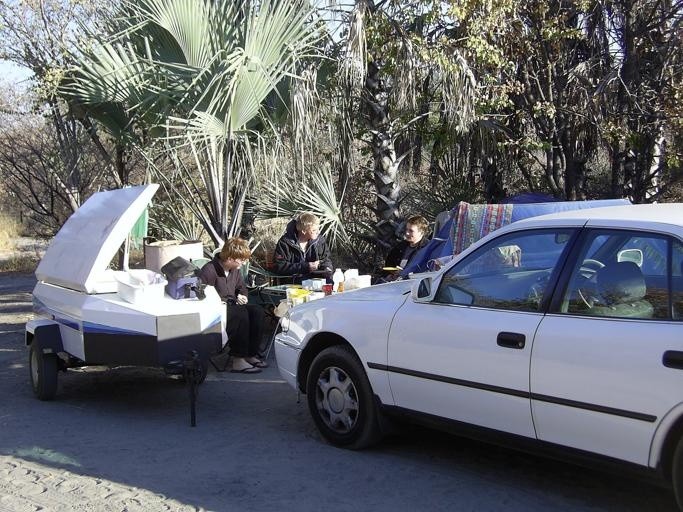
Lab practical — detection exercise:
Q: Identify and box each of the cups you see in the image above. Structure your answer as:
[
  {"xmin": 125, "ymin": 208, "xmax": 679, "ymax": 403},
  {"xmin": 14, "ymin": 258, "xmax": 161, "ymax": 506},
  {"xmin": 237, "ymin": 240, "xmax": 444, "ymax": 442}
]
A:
[{"xmin": 321, "ymin": 283, "xmax": 333, "ymax": 296}]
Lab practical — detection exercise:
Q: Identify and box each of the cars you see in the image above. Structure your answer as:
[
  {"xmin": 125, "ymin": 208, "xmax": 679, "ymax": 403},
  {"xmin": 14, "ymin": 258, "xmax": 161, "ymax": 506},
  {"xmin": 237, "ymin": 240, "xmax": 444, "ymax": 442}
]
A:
[{"xmin": 270, "ymin": 202, "xmax": 683, "ymax": 512}]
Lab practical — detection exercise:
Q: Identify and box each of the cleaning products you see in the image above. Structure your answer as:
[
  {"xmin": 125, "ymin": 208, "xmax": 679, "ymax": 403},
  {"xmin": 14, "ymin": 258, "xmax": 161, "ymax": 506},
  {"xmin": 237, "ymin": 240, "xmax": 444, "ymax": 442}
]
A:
[{"xmin": 333, "ymin": 267, "xmax": 344, "ymax": 291}]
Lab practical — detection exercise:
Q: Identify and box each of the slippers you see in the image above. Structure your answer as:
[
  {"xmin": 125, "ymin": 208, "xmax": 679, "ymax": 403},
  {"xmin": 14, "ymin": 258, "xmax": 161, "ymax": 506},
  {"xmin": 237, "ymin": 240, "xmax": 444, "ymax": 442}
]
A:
[
  {"xmin": 247, "ymin": 356, "xmax": 269, "ymax": 368},
  {"xmin": 229, "ymin": 364, "xmax": 263, "ymax": 374}
]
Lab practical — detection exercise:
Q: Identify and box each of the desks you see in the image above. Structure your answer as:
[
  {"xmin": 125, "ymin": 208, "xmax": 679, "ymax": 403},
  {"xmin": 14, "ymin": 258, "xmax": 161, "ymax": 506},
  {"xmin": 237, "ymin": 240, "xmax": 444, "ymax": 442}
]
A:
[{"xmin": 260, "ymin": 284, "xmax": 337, "ymax": 362}]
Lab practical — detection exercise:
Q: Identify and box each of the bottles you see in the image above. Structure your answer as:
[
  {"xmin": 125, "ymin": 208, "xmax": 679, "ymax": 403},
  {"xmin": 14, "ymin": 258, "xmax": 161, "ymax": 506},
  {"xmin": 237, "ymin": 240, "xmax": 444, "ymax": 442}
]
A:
[
  {"xmin": 332, "ymin": 268, "xmax": 345, "ymax": 295},
  {"xmin": 337, "ymin": 281, "xmax": 344, "ymax": 292}
]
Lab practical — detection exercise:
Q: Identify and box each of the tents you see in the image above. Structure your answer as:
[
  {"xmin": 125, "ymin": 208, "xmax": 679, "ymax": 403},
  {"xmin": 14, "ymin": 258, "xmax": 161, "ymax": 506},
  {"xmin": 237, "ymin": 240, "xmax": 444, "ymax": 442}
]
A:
[{"xmin": 397, "ymin": 191, "xmax": 682, "ymax": 279}]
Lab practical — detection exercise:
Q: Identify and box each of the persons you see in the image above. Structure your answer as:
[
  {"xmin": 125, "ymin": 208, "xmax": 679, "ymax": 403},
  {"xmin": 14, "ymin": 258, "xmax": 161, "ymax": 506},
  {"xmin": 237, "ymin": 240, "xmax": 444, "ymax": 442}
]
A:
[
  {"xmin": 270, "ymin": 212, "xmax": 334, "ymax": 287},
  {"xmin": 373, "ymin": 216, "xmax": 430, "ymax": 286},
  {"xmin": 200, "ymin": 237, "xmax": 269, "ymax": 373}
]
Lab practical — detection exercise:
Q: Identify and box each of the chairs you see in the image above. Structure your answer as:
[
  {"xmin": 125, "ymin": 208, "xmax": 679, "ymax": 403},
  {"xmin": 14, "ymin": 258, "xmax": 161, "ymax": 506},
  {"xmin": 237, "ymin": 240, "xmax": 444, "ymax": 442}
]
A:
[{"xmin": 585, "ymin": 261, "xmax": 655, "ymax": 320}]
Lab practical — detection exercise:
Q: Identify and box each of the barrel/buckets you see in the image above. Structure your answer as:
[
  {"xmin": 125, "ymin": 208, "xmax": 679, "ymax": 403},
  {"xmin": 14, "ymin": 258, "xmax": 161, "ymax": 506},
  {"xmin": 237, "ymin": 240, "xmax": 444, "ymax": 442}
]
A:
[{"xmin": 144, "ymin": 237, "xmax": 205, "ymax": 272}]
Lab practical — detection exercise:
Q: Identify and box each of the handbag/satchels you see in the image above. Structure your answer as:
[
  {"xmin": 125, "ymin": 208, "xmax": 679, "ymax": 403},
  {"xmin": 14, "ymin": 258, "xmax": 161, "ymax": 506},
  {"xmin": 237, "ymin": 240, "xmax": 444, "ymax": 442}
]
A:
[{"xmin": 159, "ymin": 256, "xmax": 207, "ymax": 300}]
